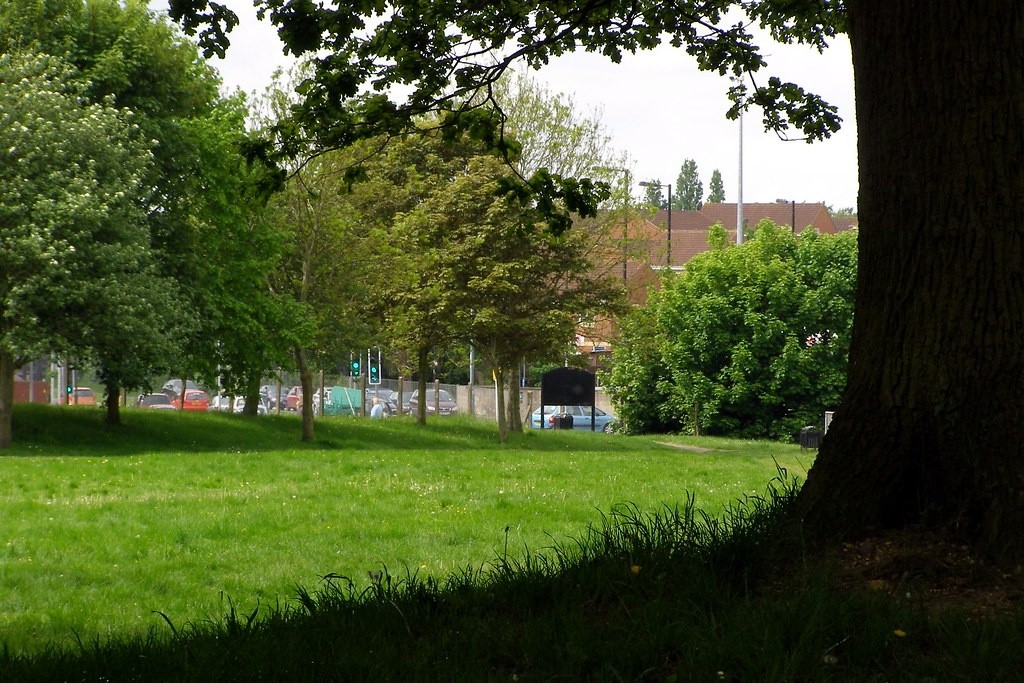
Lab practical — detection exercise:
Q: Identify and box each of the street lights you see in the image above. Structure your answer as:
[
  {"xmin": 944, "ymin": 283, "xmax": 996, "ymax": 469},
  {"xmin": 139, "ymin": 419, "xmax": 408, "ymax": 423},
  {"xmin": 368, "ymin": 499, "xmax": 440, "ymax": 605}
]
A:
[
  {"xmin": 591, "ymin": 165, "xmax": 630, "ymax": 339},
  {"xmin": 639, "ymin": 180, "xmax": 672, "ymax": 277}
]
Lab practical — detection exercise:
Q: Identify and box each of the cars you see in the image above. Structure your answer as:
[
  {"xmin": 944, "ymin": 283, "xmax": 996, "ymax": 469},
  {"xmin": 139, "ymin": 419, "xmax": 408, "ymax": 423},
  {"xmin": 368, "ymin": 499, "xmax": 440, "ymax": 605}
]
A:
[
  {"xmin": 387, "ymin": 391, "xmax": 414, "ymax": 416},
  {"xmin": 133, "ymin": 379, "xmax": 333, "ymax": 415},
  {"xmin": 365, "ymin": 387, "xmax": 394, "ymax": 415},
  {"xmin": 529, "ymin": 402, "xmax": 618, "ymax": 436},
  {"xmin": 410, "ymin": 388, "xmax": 460, "ymax": 417},
  {"xmin": 60, "ymin": 386, "xmax": 95, "ymax": 406}
]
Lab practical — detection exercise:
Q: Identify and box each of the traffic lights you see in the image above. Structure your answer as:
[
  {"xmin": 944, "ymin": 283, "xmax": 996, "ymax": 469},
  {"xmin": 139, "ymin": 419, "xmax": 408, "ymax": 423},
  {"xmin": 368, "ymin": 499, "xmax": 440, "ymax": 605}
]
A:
[
  {"xmin": 350, "ymin": 350, "xmax": 361, "ymax": 379},
  {"xmin": 367, "ymin": 348, "xmax": 381, "ymax": 385}
]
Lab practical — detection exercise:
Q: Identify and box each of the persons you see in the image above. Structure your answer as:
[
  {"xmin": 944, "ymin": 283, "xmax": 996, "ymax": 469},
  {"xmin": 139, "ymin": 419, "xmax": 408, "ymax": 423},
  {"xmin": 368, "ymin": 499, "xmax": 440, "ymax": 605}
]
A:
[{"xmin": 371, "ymin": 398, "xmax": 383, "ymax": 420}]
